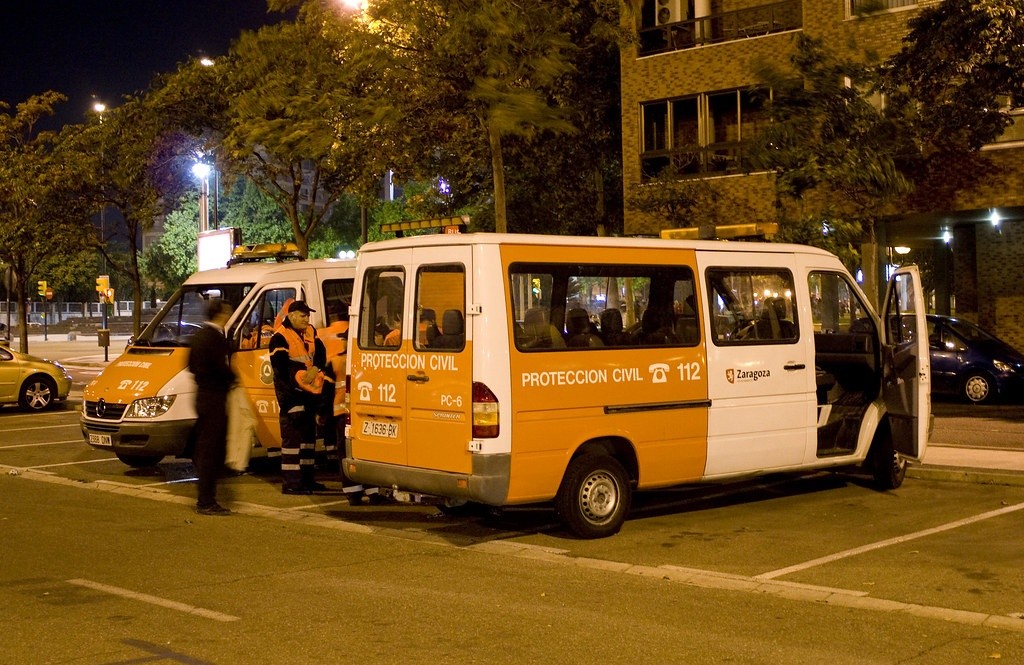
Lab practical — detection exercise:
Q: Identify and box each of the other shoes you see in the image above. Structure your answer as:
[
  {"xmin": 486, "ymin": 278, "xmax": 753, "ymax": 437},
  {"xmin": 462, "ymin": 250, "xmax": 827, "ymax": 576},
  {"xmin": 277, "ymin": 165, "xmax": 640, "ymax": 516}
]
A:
[
  {"xmin": 281, "ymin": 482, "xmax": 314, "ymax": 495},
  {"xmin": 300, "ymin": 479, "xmax": 326, "ymax": 490},
  {"xmin": 347, "ymin": 495, "xmax": 366, "ymax": 505},
  {"xmin": 196, "ymin": 503, "xmax": 231, "ymax": 515},
  {"xmin": 370, "ymin": 494, "xmax": 391, "ymax": 505}
]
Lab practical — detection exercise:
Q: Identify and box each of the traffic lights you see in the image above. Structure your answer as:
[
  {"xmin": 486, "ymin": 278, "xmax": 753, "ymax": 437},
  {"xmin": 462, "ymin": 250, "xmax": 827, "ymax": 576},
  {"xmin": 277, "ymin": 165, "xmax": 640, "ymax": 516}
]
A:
[
  {"xmin": 95, "ymin": 275, "xmax": 109, "ymax": 303},
  {"xmin": 38, "ymin": 281, "xmax": 47, "ymax": 296}
]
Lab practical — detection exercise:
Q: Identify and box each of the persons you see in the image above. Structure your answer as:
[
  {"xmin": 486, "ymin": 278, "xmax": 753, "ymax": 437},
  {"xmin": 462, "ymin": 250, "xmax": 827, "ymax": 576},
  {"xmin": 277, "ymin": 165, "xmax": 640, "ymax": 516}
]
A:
[
  {"xmin": 189, "ymin": 299, "xmax": 236, "ymax": 517},
  {"xmin": 269, "ymin": 302, "xmax": 329, "ymax": 496},
  {"xmin": 384, "ymin": 302, "xmax": 440, "ymax": 348},
  {"xmin": 213, "ymin": 292, "xmax": 533, "ymax": 509}
]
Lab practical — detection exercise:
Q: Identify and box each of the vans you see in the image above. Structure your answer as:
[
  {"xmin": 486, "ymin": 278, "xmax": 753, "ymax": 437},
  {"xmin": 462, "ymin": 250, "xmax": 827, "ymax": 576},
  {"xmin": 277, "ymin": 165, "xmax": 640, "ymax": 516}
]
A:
[
  {"xmin": 341, "ymin": 233, "xmax": 931, "ymax": 538},
  {"xmin": 79, "ymin": 258, "xmax": 466, "ymax": 475}
]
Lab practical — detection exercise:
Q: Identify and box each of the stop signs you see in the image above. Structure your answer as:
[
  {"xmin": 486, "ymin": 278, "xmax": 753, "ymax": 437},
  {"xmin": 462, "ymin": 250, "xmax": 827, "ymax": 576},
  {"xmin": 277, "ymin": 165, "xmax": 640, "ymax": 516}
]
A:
[{"xmin": 45, "ymin": 287, "xmax": 54, "ymax": 300}]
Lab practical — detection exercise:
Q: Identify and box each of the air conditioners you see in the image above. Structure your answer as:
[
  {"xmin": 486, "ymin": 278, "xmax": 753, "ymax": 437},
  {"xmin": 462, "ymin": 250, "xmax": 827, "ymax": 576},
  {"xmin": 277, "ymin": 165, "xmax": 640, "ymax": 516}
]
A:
[{"xmin": 655, "ymin": 0, "xmax": 688, "ymax": 25}]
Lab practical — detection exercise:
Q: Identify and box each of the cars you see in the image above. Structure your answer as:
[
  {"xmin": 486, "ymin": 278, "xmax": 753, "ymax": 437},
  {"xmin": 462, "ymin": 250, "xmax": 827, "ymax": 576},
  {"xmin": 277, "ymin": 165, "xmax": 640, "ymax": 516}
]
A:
[
  {"xmin": 0, "ymin": 346, "xmax": 73, "ymax": 412},
  {"xmin": 27, "ymin": 323, "xmax": 42, "ymax": 327},
  {"xmin": 124, "ymin": 321, "xmax": 203, "ymax": 352},
  {"xmin": 849, "ymin": 313, "xmax": 1024, "ymax": 405}
]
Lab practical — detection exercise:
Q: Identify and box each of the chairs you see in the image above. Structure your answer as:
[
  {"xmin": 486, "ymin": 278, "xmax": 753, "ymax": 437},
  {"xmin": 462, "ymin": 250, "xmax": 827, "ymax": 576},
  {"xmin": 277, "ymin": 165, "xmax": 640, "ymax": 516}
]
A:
[
  {"xmin": 257, "ymin": 301, "xmax": 274, "ymax": 322},
  {"xmin": 274, "ymin": 298, "xmax": 295, "ymax": 331},
  {"xmin": 421, "ymin": 298, "xmax": 795, "ymax": 350}
]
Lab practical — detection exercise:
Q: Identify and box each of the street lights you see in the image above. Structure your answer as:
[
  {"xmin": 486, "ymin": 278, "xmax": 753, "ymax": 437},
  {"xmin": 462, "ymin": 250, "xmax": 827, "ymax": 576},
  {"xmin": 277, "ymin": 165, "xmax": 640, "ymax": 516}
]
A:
[{"xmin": 885, "ymin": 235, "xmax": 912, "ymax": 343}]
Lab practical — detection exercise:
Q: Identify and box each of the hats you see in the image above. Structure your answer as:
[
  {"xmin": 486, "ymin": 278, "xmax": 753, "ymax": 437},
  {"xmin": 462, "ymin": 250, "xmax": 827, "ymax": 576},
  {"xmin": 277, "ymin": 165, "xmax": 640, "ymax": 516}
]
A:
[
  {"xmin": 288, "ymin": 301, "xmax": 316, "ymax": 313},
  {"xmin": 336, "ymin": 328, "xmax": 349, "ymax": 340},
  {"xmin": 375, "ymin": 323, "xmax": 389, "ymax": 336}
]
